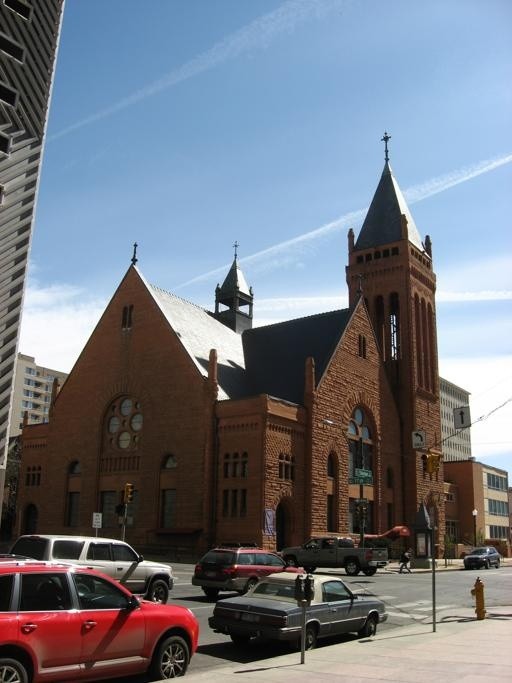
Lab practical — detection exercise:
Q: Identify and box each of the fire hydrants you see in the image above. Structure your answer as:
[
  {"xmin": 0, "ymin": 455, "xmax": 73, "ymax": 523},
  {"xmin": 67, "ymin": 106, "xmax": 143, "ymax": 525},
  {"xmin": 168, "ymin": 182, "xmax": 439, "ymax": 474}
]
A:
[{"xmin": 474, "ymin": 576, "xmax": 486, "ymax": 620}]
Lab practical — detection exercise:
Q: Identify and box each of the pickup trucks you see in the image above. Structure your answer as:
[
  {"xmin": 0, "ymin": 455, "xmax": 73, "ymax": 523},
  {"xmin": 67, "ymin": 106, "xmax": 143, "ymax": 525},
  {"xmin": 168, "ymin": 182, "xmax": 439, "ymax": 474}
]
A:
[{"xmin": 282, "ymin": 536, "xmax": 390, "ymax": 576}]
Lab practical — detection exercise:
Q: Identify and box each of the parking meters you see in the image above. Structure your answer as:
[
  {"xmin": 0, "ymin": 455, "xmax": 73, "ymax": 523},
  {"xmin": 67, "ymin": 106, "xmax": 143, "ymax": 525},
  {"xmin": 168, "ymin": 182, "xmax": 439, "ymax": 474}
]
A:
[{"xmin": 294, "ymin": 574, "xmax": 315, "ymax": 664}]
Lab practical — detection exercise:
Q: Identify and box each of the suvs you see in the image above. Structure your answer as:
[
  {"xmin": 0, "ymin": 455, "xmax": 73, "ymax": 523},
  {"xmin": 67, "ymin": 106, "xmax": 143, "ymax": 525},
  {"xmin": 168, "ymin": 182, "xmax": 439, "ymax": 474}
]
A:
[
  {"xmin": 191, "ymin": 545, "xmax": 308, "ymax": 599},
  {"xmin": 8, "ymin": 535, "xmax": 174, "ymax": 607},
  {"xmin": 0, "ymin": 554, "xmax": 200, "ymax": 683}
]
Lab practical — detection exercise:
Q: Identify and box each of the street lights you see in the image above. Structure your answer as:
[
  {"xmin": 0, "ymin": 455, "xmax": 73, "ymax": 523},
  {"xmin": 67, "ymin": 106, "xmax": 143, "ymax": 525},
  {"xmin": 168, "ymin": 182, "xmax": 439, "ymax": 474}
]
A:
[{"xmin": 472, "ymin": 508, "xmax": 478, "ymax": 546}]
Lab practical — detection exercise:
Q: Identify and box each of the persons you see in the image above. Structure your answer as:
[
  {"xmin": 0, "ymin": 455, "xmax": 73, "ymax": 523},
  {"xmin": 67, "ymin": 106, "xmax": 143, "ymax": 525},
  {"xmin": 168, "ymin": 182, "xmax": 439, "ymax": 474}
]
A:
[{"xmin": 398, "ymin": 548, "xmax": 412, "ymax": 574}]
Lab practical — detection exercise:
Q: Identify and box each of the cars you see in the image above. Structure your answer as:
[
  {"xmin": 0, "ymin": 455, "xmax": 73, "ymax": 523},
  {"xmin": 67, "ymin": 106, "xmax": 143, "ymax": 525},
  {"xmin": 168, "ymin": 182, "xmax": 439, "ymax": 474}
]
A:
[
  {"xmin": 206, "ymin": 575, "xmax": 389, "ymax": 652},
  {"xmin": 464, "ymin": 546, "xmax": 501, "ymax": 569}
]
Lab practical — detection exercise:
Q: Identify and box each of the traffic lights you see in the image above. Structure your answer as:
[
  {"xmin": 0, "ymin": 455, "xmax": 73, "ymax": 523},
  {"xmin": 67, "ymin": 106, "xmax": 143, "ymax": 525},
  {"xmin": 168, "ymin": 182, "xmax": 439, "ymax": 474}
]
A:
[
  {"xmin": 421, "ymin": 452, "xmax": 440, "ymax": 473},
  {"xmin": 124, "ymin": 483, "xmax": 135, "ymax": 504}
]
